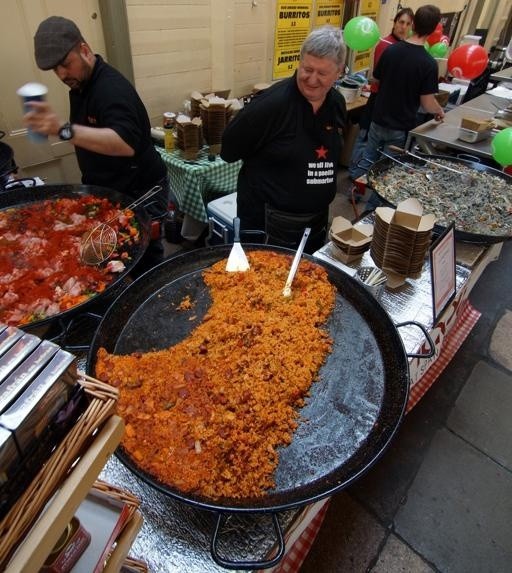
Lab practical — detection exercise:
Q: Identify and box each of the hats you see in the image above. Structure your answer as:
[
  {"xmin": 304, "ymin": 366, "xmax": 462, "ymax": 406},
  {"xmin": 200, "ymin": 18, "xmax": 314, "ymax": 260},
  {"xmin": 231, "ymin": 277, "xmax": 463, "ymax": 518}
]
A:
[{"xmin": 34, "ymin": 16, "xmax": 81, "ymax": 71}]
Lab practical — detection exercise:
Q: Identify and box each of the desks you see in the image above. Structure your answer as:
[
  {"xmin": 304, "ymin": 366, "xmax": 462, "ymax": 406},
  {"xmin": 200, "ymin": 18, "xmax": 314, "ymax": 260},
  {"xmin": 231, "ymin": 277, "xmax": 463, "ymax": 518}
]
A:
[
  {"xmin": 143, "ymin": 95, "xmax": 369, "ymax": 243},
  {"xmin": 405, "ymin": 79, "xmax": 511, "ymax": 173}
]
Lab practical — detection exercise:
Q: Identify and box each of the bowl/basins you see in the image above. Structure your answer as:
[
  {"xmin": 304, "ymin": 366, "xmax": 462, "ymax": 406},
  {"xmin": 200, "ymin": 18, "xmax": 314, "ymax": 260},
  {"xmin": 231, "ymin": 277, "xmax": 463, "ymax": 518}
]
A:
[{"xmin": 339, "ymin": 86, "xmax": 357, "ymax": 103}]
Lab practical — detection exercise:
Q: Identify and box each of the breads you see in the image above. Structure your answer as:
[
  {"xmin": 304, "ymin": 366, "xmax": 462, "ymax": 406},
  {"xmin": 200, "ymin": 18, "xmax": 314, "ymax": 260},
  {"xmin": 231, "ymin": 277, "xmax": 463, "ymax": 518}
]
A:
[{"xmin": 461, "ymin": 117, "xmax": 490, "ymax": 132}]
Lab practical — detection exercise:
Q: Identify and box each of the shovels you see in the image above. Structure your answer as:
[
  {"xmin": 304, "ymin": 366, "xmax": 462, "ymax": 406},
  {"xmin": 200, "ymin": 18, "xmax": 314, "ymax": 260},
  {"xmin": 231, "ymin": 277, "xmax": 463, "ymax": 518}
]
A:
[{"xmin": 225, "ymin": 217, "xmax": 251, "ymax": 273}]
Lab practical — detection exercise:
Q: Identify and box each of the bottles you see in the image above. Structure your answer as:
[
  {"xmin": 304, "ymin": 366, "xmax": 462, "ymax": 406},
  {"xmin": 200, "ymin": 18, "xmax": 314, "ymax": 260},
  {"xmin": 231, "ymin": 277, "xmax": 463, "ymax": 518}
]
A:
[{"xmin": 164, "ymin": 112, "xmax": 176, "ymax": 154}]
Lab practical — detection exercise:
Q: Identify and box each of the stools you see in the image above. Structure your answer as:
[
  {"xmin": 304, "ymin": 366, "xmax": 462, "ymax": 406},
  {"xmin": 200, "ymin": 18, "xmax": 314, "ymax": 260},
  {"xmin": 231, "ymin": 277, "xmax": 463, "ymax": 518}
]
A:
[{"xmin": 203, "ymin": 189, "xmax": 241, "ymax": 251}]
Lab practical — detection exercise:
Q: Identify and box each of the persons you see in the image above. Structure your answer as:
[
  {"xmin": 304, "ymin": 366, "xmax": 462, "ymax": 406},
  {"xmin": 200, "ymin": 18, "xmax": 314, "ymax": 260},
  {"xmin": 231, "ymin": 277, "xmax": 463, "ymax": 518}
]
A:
[
  {"xmin": 348, "ymin": 6, "xmax": 415, "ymax": 178},
  {"xmin": 346, "ymin": 3, "xmax": 445, "ymax": 208},
  {"xmin": 218, "ymin": 25, "xmax": 350, "ymax": 253},
  {"xmin": 23, "ymin": 12, "xmax": 170, "ymax": 282}
]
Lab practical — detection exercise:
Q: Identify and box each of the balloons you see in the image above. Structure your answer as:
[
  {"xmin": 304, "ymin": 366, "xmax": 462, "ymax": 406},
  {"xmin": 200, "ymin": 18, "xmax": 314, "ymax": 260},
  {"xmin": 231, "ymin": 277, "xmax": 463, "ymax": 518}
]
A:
[
  {"xmin": 343, "ymin": 15, "xmax": 380, "ymax": 52},
  {"xmin": 446, "ymin": 43, "xmax": 489, "ymax": 81},
  {"xmin": 424, "ymin": 21, "xmax": 448, "ymax": 58},
  {"xmin": 490, "ymin": 126, "xmax": 512, "ymax": 170}
]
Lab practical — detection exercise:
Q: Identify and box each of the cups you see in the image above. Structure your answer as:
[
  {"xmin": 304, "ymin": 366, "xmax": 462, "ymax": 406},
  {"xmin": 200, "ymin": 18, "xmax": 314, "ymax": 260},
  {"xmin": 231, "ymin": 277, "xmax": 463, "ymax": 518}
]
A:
[{"xmin": 15, "ymin": 83, "xmax": 49, "ymax": 145}]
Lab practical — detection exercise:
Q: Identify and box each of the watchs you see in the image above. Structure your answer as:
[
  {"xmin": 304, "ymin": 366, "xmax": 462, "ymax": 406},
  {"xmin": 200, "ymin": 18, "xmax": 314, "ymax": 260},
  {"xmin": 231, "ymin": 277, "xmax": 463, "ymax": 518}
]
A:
[{"xmin": 58, "ymin": 120, "xmax": 74, "ymax": 142}]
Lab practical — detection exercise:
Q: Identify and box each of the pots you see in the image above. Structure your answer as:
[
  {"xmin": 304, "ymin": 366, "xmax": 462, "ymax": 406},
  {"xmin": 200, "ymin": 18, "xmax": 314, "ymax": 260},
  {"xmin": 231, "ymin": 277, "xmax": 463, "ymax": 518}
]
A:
[
  {"xmin": 95, "ymin": 241, "xmax": 408, "ymax": 513},
  {"xmin": 4, "ymin": 181, "xmax": 145, "ymax": 330}
]
